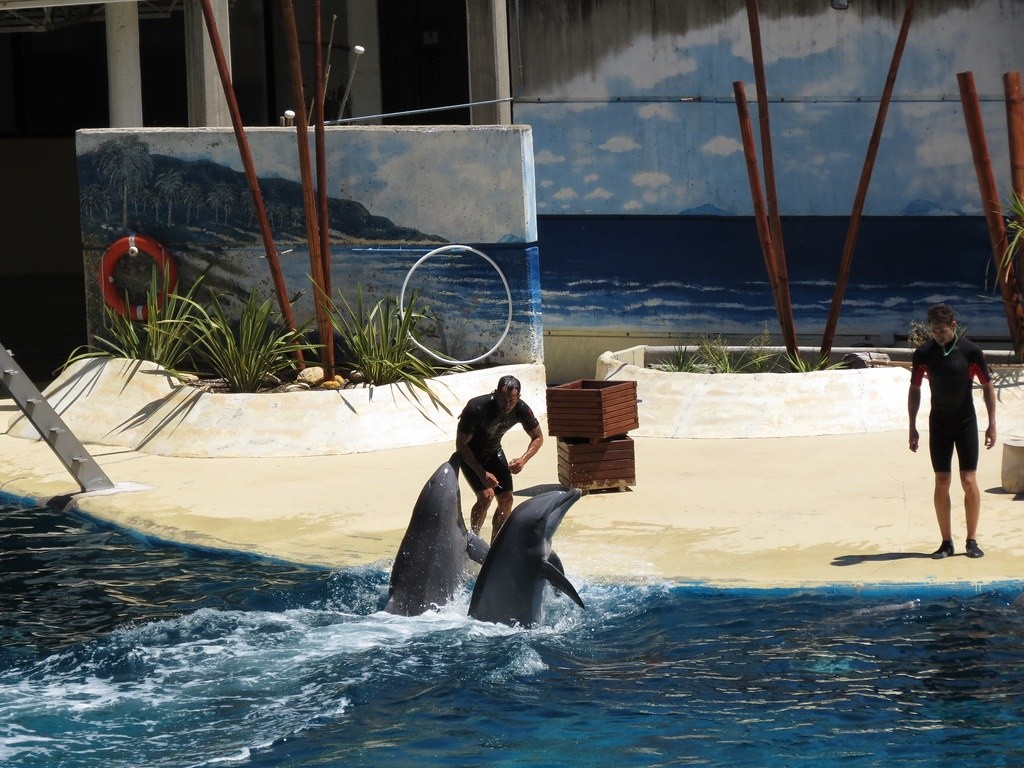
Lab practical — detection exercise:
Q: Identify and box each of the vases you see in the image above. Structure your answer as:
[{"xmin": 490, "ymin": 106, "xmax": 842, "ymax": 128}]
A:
[
  {"xmin": 546, "ymin": 378, "xmax": 639, "ymax": 446},
  {"xmin": 557, "ymin": 433, "xmax": 636, "ymax": 497}
]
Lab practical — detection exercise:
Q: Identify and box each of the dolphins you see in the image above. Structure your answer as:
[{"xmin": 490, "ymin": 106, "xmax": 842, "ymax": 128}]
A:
[
  {"xmin": 383, "ymin": 452, "xmax": 490, "ymax": 618},
  {"xmin": 466, "ymin": 488, "xmax": 585, "ymax": 628}
]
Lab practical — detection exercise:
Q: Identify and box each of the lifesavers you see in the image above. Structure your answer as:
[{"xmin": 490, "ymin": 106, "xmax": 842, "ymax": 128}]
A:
[{"xmin": 96, "ymin": 236, "xmax": 175, "ymax": 321}]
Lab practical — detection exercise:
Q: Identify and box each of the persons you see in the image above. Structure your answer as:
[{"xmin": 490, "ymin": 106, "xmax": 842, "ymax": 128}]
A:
[
  {"xmin": 909, "ymin": 302, "xmax": 996, "ymax": 559},
  {"xmin": 457, "ymin": 377, "xmax": 543, "ymax": 547}
]
